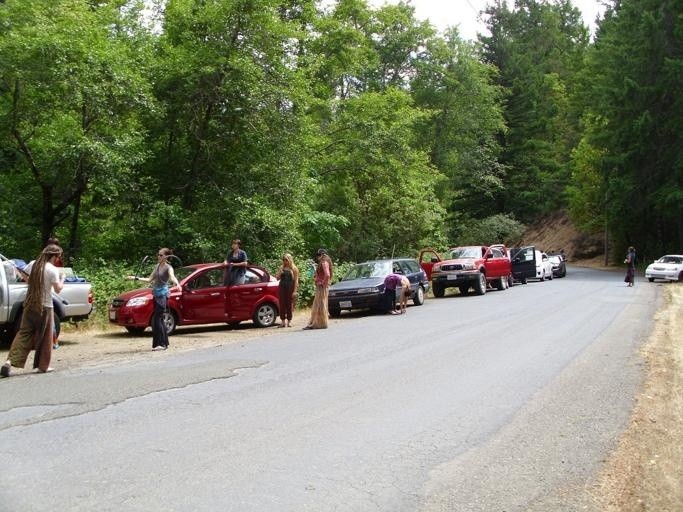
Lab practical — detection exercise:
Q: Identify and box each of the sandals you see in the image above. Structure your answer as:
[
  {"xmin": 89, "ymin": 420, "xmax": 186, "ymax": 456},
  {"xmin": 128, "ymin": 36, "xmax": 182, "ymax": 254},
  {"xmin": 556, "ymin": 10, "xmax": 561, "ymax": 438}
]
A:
[{"xmin": 0, "ymin": 364, "xmax": 10, "ymax": 377}]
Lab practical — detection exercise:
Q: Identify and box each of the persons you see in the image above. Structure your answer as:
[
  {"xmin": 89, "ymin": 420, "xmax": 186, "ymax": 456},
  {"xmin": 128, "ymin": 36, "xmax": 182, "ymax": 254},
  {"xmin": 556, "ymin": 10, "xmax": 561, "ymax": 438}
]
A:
[
  {"xmin": 223, "ymin": 240, "xmax": 247, "ymax": 287},
  {"xmin": 0, "ymin": 242, "xmax": 66, "ymax": 375},
  {"xmin": 302, "ymin": 247, "xmax": 331, "ymax": 329},
  {"xmin": 125, "ymin": 247, "xmax": 181, "ymax": 352},
  {"xmin": 48, "ymin": 237, "xmax": 62, "ymax": 269},
  {"xmin": 623, "ymin": 247, "xmax": 637, "ymax": 288},
  {"xmin": 273, "ymin": 252, "xmax": 299, "ymax": 329},
  {"xmin": 383, "ymin": 273, "xmax": 412, "ymax": 315},
  {"xmin": 507, "ymin": 247, "xmax": 567, "ymax": 285}
]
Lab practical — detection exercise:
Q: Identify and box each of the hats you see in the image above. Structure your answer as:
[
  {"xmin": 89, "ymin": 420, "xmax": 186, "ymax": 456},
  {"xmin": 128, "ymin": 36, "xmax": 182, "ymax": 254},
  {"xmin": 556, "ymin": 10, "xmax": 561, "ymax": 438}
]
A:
[{"xmin": 315, "ymin": 249, "xmax": 327, "ymax": 255}]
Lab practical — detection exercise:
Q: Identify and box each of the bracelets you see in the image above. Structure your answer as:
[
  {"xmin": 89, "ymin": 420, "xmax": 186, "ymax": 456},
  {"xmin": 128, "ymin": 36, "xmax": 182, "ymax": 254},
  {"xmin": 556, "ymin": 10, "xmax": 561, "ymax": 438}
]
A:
[{"xmin": 133, "ymin": 276, "xmax": 138, "ymax": 281}]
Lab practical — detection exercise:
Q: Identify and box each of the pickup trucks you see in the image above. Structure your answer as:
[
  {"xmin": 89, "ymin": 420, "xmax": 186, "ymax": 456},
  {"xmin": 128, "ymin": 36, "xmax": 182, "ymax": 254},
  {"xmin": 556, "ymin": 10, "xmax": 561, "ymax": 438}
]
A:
[{"xmin": 0, "ymin": 257, "xmax": 92, "ymax": 350}]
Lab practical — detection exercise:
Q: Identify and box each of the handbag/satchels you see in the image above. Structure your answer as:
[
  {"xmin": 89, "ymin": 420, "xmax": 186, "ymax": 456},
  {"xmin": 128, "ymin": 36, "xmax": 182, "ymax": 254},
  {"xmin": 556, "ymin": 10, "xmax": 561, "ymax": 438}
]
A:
[{"xmin": 153, "ymin": 286, "xmax": 168, "ymax": 297}]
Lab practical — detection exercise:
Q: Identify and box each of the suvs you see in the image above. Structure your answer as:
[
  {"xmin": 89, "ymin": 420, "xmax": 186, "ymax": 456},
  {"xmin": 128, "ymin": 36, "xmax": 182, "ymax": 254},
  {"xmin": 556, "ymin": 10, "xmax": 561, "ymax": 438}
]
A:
[{"xmin": 419, "ymin": 245, "xmax": 511, "ymax": 296}]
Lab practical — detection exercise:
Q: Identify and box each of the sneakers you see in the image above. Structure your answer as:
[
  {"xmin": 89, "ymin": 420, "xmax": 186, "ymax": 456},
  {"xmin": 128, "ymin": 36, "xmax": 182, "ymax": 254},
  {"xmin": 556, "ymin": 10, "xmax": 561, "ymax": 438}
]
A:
[{"xmin": 151, "ymin": 346, "xmax": 165, "ymax": 350}]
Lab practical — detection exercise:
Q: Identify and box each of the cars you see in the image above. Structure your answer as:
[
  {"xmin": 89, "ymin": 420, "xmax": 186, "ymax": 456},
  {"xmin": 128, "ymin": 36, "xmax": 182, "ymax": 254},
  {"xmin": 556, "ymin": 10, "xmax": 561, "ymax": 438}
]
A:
[
  {"xmin": 489, "ymin": 246, "xmax": 567, "ymax": 292},
  {"xmin": 645, "ymin": 255, "xmax": 683, "ymax": 282},
  {"xmin": 108, "ymin": 263, "xmax": 298, "ymax": 335},
  {"xmin": 327, "ymin": 258, "xmax": 429, "ymax": 315}
]
